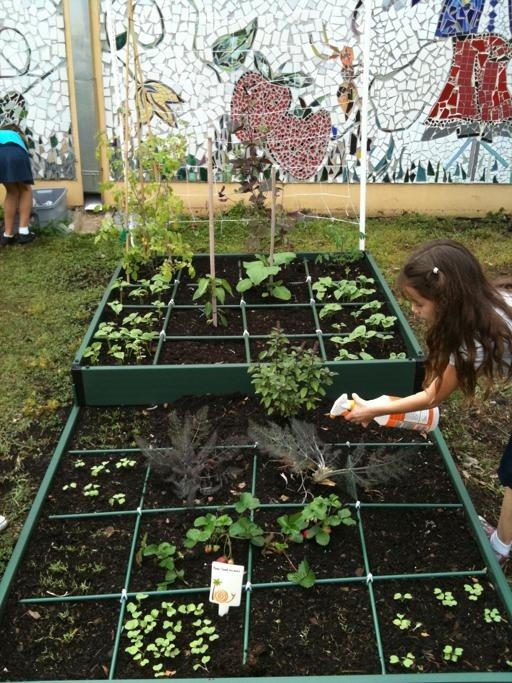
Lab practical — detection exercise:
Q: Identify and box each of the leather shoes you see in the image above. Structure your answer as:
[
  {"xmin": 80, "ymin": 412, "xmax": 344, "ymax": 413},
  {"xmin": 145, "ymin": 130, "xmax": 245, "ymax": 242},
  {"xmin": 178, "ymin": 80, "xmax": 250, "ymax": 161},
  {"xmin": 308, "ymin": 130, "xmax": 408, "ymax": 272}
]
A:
[
  {"xmin": 1, "ymin": 233, "xmax": 16, "ymax": 245},
  {"xmin": 18, "ymin": 231, "xmax": 37, "ymax": 244}
]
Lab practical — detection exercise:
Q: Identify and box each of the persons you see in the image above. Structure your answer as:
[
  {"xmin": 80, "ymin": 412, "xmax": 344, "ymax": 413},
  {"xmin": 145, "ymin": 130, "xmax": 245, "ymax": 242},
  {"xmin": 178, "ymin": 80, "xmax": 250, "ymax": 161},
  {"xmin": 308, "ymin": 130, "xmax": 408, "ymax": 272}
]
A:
[
  {"xmin": 0, "ymin": 122, "xmax": 37, "ymax": 245},
  {"xmin": 343, "ymin": 238, "xmax": 511, "ymax": 576}
]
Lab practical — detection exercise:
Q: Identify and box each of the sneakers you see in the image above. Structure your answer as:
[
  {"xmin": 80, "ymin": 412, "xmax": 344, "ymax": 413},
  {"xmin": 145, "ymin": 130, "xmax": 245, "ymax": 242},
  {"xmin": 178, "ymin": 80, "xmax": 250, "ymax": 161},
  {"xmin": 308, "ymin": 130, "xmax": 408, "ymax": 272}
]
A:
[{"xmin": 477, "ymin": 515, "xmax": 507, "ymax": 568}]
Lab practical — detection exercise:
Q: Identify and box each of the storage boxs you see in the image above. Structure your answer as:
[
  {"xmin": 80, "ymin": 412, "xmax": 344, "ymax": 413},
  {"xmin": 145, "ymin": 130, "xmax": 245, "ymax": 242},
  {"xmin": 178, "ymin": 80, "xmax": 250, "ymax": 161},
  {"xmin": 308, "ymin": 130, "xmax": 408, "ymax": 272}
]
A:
[{"xmin": 13, "ymin": 187, "xmax": 69, "ymax": 234}]
